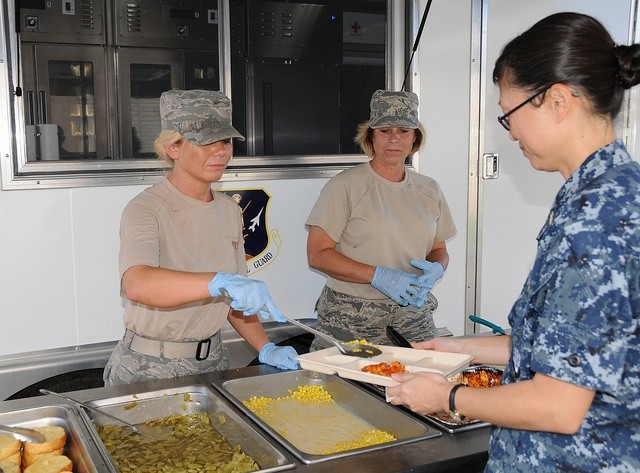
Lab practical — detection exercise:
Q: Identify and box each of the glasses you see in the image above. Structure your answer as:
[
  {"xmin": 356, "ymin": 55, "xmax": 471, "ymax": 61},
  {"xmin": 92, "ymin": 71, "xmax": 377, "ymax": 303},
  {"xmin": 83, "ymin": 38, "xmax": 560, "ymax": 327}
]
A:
[{"xmin": 497, "ymin": 88, "xmax": 547, "ymax": 131}]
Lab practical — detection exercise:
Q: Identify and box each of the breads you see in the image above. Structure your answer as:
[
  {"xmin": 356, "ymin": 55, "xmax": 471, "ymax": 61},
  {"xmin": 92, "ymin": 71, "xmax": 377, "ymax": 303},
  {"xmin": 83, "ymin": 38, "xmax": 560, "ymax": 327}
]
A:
[{"xmin": 0, "ymin": 422, "xmax": 77, "ymax": 473}]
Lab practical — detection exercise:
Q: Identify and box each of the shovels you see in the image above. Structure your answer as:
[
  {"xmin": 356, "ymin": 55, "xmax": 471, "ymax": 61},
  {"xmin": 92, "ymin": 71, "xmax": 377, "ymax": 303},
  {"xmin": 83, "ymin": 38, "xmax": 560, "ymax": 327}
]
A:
[{"xmin": 260, "ymin": 306, "xmax": 382, "ymax": 358}]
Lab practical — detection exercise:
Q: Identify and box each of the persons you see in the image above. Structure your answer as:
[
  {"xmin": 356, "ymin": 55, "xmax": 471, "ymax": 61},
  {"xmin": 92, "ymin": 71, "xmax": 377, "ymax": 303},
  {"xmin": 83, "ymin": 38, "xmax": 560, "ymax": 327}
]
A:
[
  {"xmin": 303, "ymin": 87, "xmax": 459, "ymax": 350},
  {"xmin": 385, "ymin": 12, "xmax": 640, "ymax": 473},
  {"xmin": 102, "ymin": 88, "xmax": 301, "ymax": 385}
]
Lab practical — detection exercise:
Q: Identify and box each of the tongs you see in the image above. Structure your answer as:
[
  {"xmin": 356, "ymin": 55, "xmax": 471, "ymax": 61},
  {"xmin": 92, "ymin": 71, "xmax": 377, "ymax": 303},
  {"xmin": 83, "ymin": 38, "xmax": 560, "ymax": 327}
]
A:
[{"xmin": 1, "ymin": 424, "xmax": 47, "ymax": 444}]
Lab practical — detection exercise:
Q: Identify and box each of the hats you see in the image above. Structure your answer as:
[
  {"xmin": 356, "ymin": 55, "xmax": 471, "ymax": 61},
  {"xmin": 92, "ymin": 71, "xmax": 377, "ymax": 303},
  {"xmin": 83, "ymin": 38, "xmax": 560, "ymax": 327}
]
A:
[
  {"xmin": 369, "ymin": 89, "xmax": 419, "ymax": 130},
  {"xmin": 159, "ymin": 90, "xmax": 246, "ymax": 146}
]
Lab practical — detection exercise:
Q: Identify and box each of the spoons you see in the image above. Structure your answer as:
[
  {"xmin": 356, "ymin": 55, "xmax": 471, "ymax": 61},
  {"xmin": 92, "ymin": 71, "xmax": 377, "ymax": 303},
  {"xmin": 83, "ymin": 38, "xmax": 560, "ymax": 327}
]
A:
[{"xmin": 221, "ymin": 288, "xmax": 381, "ymax": 357}]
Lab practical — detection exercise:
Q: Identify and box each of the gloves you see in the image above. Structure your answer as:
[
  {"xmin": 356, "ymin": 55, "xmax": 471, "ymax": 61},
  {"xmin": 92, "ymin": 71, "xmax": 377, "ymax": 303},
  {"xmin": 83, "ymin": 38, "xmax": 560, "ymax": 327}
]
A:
[
  {"xmin": 208, "ymin": 272, "xmax": 287, "ymax": 323},
  {"xmin": 258, "ymin": 343, "xmax": 300, "ymax": 370},
  {"xmin": 410, "ymin": 259, "xmax": 444, "ymax": 308},
  {"xmin": 371, "ymin": 266, "xmax": 433, "ymax": 306}
]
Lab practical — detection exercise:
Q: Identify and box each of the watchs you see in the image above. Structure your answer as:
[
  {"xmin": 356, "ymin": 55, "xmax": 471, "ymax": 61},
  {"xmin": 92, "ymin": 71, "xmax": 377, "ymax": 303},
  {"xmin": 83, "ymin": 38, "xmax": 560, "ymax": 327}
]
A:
[{"xmin": 449, "ymin": 383, "xmax": 469, "ymax": 420}]
